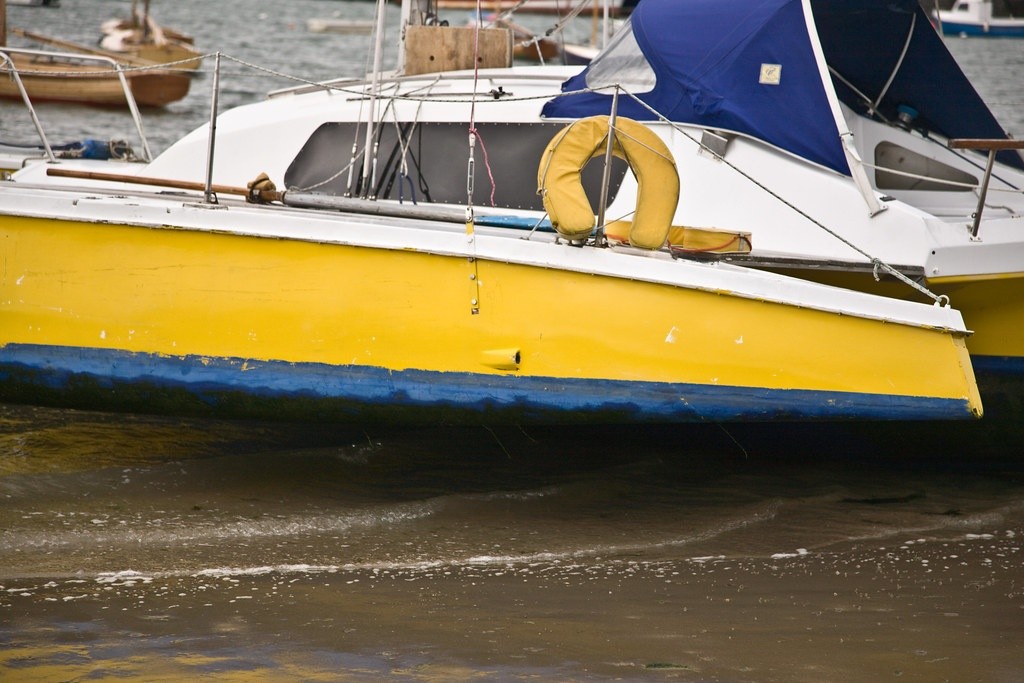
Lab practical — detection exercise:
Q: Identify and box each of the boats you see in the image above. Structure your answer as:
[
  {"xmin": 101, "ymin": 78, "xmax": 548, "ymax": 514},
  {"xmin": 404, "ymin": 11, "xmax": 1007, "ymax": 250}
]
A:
[
  {"xmin": 0, "ymin": 0, "xmax": 201, "ymax": 109},
  {"xmin": 931, "ymin": 0, "xmax": 1024, "ymax": 38}
]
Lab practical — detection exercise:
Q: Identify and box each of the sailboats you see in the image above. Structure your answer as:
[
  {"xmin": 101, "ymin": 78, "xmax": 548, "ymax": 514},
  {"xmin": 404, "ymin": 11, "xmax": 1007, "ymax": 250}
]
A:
[{"xmin": 0, "ymin": 0, "xmax": 1024, "ymax": 432}]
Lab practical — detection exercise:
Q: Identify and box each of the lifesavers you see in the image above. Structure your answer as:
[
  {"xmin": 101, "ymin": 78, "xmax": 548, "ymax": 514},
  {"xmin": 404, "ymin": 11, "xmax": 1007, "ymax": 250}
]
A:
[{"xmin": 535, "ymin": 111, "xmax": 682, "ymax": 250}]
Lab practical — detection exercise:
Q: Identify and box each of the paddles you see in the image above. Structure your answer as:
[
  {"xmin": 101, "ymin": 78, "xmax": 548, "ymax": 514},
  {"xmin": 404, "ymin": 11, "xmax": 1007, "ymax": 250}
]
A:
[
  {"xmin": 45, "ymin": 165, "xmax": 751, "ymax": 256},
  {"xmin": 9, "ymin": 27, "xmax": 204, "ymax": 80}
]
